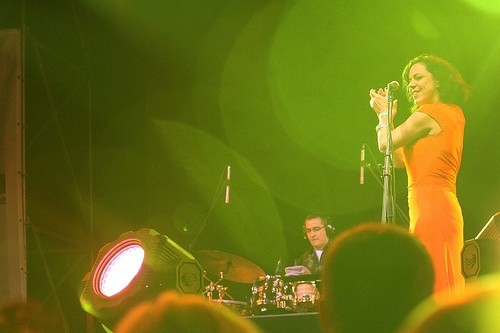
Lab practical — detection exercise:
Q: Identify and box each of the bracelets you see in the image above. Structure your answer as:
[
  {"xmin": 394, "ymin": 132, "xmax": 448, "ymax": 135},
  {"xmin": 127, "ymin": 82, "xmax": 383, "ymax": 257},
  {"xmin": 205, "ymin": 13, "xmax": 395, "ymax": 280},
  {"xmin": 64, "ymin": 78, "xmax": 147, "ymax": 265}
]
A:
[
  {"xmin": 376, "ymin": 122, "xmax": 387, "ymax": 130},
  {"xmin": 379, "ymin": 111, "xmax": 387, "ymax": 120}
]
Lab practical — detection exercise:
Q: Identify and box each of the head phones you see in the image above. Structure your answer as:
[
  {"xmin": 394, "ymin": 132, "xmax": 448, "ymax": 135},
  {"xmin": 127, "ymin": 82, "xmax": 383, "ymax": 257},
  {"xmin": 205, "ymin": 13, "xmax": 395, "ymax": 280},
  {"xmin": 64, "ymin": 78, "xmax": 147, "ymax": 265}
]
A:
[{"xmin": 302, "ymin": 216, "xmax": 333, "ymax": 240}]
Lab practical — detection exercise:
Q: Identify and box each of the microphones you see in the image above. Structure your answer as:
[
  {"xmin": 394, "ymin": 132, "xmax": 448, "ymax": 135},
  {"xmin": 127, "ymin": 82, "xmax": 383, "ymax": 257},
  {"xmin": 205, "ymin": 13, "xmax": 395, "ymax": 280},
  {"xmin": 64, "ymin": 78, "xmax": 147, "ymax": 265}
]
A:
[
  {"xmin": 360, "ymin": 145, "xmax": 365, "ymax": 185},
  {"xmin": 225, "ymin": 164, "xmax": 230, "ymax": 203},
  {"xmin": 388, "ymin": 81, "xmax": 399, "ymax": 90}
]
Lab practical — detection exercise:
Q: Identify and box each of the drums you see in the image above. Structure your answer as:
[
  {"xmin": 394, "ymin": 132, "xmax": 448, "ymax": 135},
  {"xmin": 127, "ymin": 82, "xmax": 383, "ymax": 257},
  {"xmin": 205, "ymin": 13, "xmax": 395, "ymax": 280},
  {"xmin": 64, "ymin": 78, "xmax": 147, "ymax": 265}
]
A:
[
  {"xmin": 249, "ymin": 275, "xmax": 297, "ymax": 313},
  {"xmin": 292, "ymin": 279, "xmax": 318, "ymax": 307},
  {"xmin": 211, "ymin": 298, "xmax": 248, "ymax": 317}
]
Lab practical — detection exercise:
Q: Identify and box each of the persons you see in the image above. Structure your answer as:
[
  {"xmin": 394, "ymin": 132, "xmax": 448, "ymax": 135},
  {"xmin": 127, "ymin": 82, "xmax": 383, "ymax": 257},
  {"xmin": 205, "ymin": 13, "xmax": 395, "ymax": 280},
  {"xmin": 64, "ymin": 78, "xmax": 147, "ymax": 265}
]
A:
[
  {"xmin": 316, "ymin": 222, "xmax": 435, "ymax": 333},
  {"xmin": 295, "ymin": 212, "xmax": 332, "ymax": 273},
  {"xmin": 369, "ymin": 56, "xmax": 470, "ymax": 297},
  {"xmin": 114, "ymin": 291, "xmax": 259, "ymax": 333}
]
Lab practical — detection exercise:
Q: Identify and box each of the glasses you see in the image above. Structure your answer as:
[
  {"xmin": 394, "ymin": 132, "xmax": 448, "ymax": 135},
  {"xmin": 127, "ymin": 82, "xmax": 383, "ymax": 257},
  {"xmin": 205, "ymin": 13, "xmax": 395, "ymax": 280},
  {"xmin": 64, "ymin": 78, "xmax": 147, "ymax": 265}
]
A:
[{"xmin": 306, "ymin": 226, "xmax": 325, "ymax": 233}]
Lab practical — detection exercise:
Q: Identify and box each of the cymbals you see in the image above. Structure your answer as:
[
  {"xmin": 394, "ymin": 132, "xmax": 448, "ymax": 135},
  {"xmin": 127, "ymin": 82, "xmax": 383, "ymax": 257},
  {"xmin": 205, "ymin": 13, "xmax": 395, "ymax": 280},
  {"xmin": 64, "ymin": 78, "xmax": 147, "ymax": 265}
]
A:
[{"xmin": 194, "ymin": 249, "xmax": 266, "ymax": 283}]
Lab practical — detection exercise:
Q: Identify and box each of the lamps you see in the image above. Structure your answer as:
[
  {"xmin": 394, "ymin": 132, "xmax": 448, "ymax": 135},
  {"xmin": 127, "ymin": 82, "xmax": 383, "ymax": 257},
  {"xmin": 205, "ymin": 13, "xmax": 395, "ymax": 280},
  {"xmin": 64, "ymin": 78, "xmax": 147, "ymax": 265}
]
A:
[{"xmin": 81, "ymin": 228, "xmax": 205, "ymax": 323}]
